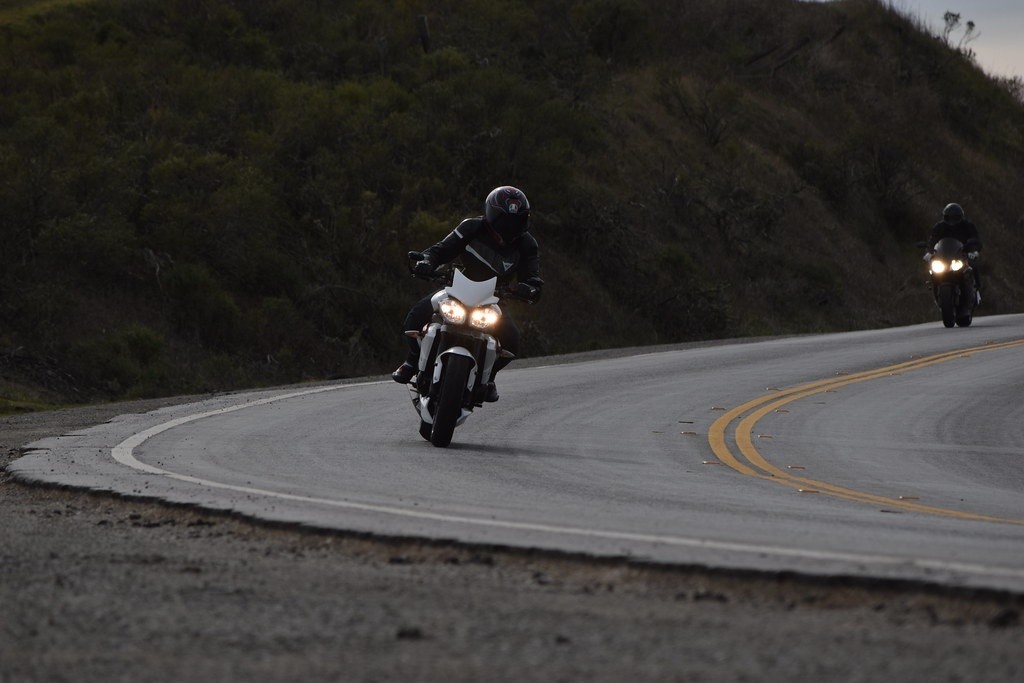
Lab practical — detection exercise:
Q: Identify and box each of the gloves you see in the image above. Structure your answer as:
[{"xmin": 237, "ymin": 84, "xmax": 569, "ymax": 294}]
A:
[
  {"xmin": 512, "ymin": 280, "xmax": 540, "ymax": 300},
  {"xmin": 968, "ymin": 252, "xmax": 977, "ymax": 260},
  {"xmin": 414, "ymin": 253, "xmax": 435, "ymax": 278}
]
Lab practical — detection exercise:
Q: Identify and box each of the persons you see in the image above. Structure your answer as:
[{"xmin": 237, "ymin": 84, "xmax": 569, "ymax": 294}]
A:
[
  {"xmin": 392, "ymin": 185, "xmax": 545, "ymax": 404},
  {"xmin": 921, "ymin": 203, "xmax": 984, "ymax": 304}
]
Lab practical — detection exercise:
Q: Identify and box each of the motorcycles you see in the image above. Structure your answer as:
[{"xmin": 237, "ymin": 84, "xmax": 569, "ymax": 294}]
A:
[
  {"xmin": 916, "ymin": 238, "xmax": 984, "ymax": 328},
  {"xmin": 394, "ymin": 250, "xmax": 541, "ymax": 449}
]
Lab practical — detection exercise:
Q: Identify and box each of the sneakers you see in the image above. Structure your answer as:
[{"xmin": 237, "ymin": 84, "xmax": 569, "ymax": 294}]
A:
[
  {"xmin": 484, "ymin": 381, "xmax": 499, "ymax": 402},
  {"xmin": 392, "ymin": 360, "xmax": 416, "ymax": 384}
]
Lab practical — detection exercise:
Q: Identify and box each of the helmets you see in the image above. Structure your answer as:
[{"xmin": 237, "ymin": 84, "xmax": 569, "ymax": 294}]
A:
[
  {"xmin": 942, "ymin": 202, "xmax": 964, "ymax": 226},
  {"xmin": 485, "ymin": 185, "xmax": 531, "ymax": 248}
]
